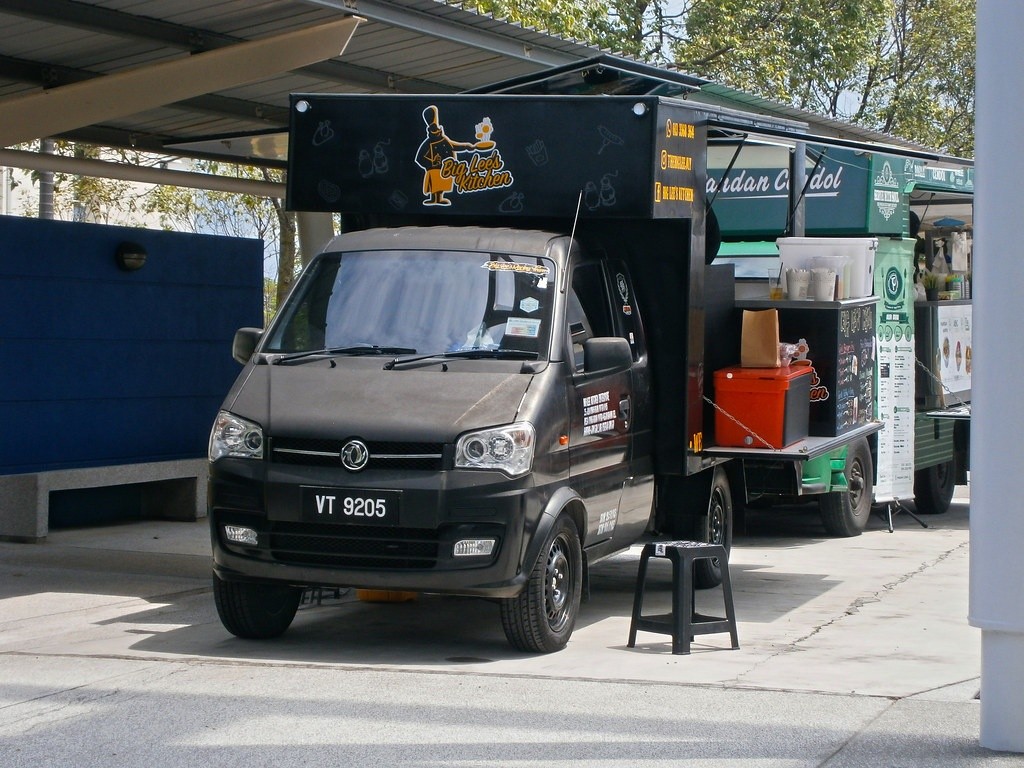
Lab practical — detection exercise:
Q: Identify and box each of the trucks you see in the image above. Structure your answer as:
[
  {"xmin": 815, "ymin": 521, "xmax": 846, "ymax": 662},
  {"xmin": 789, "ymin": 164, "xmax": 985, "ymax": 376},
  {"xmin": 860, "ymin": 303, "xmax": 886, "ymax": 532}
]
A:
[{"xmin": 207, "ymin": 93, "xmax": 975, "ymax": 653}]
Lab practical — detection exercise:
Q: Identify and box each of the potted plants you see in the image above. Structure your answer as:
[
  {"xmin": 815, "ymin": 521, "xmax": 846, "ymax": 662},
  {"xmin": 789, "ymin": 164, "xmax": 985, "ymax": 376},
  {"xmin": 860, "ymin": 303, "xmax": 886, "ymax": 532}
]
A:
[{"xmin": 922, "ymin": 272, "xmax": 941, "ymax": 301}]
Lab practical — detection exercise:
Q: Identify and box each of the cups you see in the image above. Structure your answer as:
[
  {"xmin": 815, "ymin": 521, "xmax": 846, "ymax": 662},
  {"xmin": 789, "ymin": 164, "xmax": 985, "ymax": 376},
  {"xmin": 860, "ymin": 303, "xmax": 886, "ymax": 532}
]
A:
[
  {"xmin": 767, "ymin": 268, "xmax": 783, "ymax": 300},
  {"xmin": 810, "ymin": 266, "xmax": 836, "ymax": 302},
  {"xmin": 785, "ymin": 267, "xmax": 811, "ymax": 301}
]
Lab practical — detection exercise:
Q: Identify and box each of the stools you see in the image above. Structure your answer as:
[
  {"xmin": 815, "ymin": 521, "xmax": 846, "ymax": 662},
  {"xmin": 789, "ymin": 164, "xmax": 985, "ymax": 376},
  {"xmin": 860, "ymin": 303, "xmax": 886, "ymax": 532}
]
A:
[{"xmin": 626, "ymin": 540, "xmax": 740, "ymax": 655}]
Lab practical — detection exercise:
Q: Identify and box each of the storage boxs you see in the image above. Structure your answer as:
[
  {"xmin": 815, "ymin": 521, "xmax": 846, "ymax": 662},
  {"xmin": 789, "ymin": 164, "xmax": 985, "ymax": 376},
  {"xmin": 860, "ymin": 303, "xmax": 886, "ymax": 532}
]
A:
[
  {"xmin": 775, "ymin": 237, "xmax": 879, "ymax": 297},
  {"xmin": 713, "ymin": 364, "xmax": 811, "ymax": 448}
]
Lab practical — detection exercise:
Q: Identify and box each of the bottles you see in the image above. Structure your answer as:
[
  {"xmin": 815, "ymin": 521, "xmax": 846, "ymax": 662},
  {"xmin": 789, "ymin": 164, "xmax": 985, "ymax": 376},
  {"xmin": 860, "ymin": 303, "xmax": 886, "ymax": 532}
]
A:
[{"xmin": 944, "ymin": 273, "xmax": 970, "ymax": 299}]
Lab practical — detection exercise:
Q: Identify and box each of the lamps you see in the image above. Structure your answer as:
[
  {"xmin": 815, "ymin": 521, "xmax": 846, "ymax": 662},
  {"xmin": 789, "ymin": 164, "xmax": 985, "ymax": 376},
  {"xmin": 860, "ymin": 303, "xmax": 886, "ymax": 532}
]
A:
[{"xmin": 115, "ymin": 241, "xmax": 150, "ymax": 272}]
